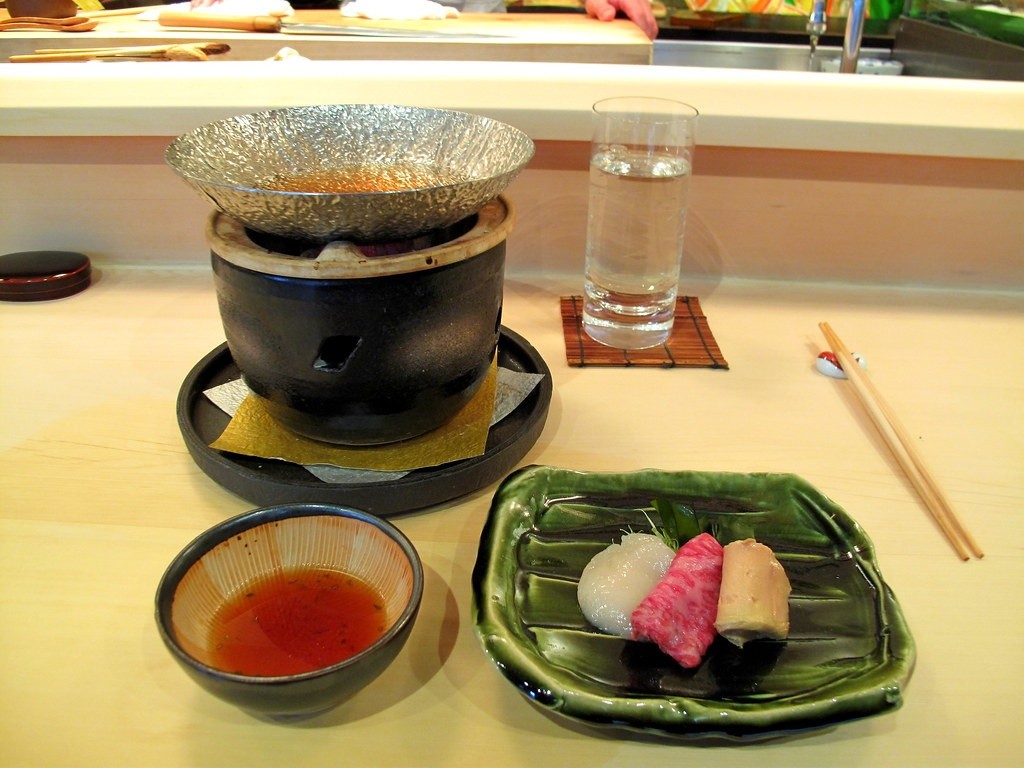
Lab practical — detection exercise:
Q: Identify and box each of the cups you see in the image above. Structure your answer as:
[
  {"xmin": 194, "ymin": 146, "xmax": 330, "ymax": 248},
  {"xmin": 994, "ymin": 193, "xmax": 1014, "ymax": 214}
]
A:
[{"xmin": 583, "ymin": 95, "xmax": 699, "ymax": 350}]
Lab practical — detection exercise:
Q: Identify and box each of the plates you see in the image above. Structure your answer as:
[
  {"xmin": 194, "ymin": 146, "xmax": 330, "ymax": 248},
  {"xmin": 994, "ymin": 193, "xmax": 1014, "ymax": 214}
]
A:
[{"xmin": 470, "ymin": 464, "xmax": 916, "ymax": 742}]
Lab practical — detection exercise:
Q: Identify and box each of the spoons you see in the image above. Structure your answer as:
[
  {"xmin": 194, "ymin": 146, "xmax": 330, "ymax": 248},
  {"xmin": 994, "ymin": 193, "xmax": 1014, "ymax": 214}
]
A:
[{"xmin": 0, "ymin": 16, "xmax": 99, "ymax": 31}]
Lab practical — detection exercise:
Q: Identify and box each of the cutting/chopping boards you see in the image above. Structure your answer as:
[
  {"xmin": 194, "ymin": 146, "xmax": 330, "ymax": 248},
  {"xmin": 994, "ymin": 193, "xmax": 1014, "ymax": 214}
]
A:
[{"xmin": 0, "ymin": 8, "xmax": 654, "ymax": 64}]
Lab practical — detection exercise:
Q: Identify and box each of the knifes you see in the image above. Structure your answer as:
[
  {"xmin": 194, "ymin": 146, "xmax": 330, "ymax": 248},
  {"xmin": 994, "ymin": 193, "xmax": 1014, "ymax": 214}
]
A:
[{"xmin": 159, "ymin": 11, "xmax": 504, "ymax": 40}]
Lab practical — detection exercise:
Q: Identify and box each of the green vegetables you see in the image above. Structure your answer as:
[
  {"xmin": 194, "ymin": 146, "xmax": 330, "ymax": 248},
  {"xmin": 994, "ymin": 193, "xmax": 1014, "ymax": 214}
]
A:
[{"xmin": 619, "ymin": 524, "xmax": 680, "ymax": 553}]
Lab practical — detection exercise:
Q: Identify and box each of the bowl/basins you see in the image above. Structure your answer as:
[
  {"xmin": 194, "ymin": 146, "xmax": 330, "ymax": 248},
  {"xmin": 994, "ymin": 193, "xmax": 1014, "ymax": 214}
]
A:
[
  {"xmin": 163, "ymin": 104, "xmax": 536, "ymax": 248},
  {"xmin": 153, "ymin": 502, "xmax": 423, "ymax": 721}
]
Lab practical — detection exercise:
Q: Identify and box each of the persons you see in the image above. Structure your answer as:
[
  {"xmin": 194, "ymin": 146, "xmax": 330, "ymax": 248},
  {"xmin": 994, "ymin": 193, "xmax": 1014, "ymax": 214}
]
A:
[{"xmin": 190, "ymin": 0, "xmax": 660, "ymax": 41}]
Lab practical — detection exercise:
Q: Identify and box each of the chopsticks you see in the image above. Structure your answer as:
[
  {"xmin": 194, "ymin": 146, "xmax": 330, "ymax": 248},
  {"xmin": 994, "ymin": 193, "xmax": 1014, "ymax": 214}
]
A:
[{"xmin": 818, "ymin": 320, "xmax": 985, "ymax": 561}]
